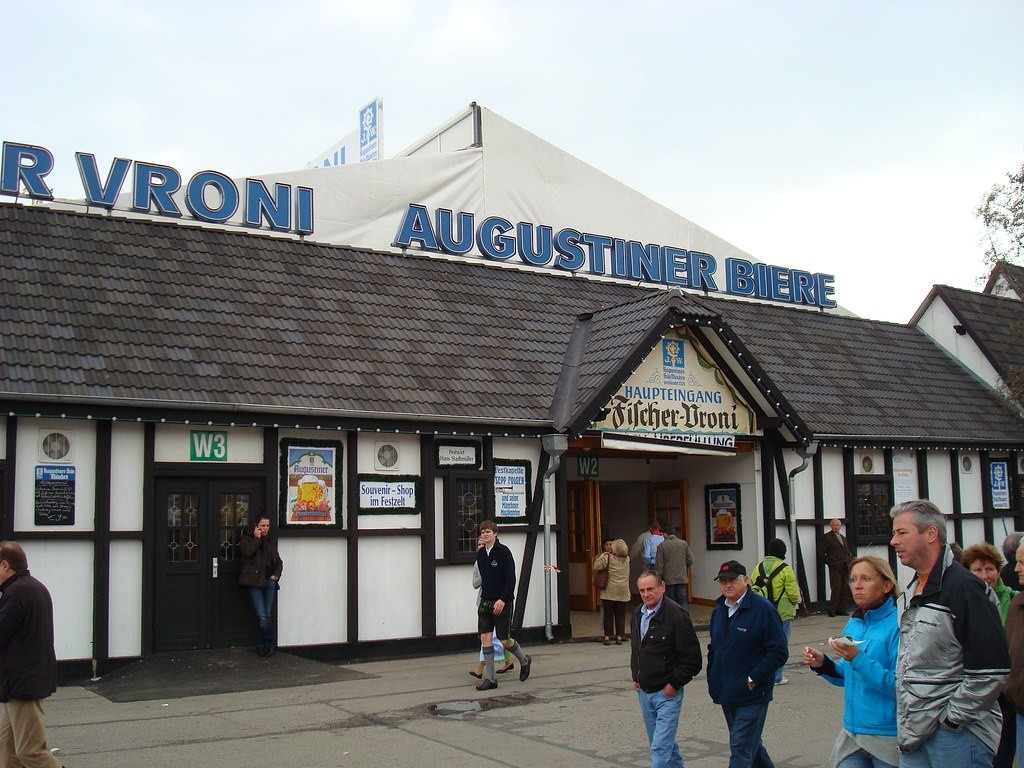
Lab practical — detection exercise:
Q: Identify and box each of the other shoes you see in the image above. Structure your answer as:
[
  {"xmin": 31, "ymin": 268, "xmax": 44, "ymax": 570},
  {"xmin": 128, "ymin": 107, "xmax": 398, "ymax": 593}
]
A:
[
  {"xmin": 469, "ymin": 672, "xmax": 483, "ymax": 679},
  {"xmin": 603, "ymin": 639, "xmax": 611, "ymax": 644},
  {"xmin": 774, "ymin": 677, "xmax": 789, "ymax": 685},
  {"xmin": 496, "ymin": 663, "xmax": 514, "ymax": 673},
  {"xmin": 828, "ymin": 611, "xmax": 836, "ymax": 617},
  {"xmin": 616, "ymin": 639, "xmax": 622, "ymax": 645},
  {"xmin": 837, "ymin": 611, "xmax": 849, "ymax": 616},
  {"xmin": 266, "ymin": 646, "xmax": 275, "ymax": 657}
]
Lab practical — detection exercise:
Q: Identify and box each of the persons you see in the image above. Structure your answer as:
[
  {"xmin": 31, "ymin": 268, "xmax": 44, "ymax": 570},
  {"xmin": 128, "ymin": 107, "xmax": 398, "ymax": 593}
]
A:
[
  {"xmin": 631, "ymin": 570, "xmax": 702, "ymax": 768},
  {"xmin": 889, "ymin": 499, "xmax": 1012, "ymax": 768},
  {"xmin": 469, "ymin": 519, "xmax": 531, "ymax": 691},
  {"xmin": 238, "ymin": 514, "xmax": 283, "ymax": 658},
  {"xmin": 750, "ymin": 538, "xmax": 801, "ymax": 686},
  {"xmin": 0, "ymin": 541, "xmax": 67, "ymax": 768},
  {"xmin": 706, "ymin": 560, "xmax": 789, "ymax": 768},
  {"xmin": 594, "ymin": 517, "xmax": 694, "ymax": 645},
  {"xmin": 822, "ymin": 519, "xmax": 852, "ymax": 617},
  {"xmin": 948, "ymin": 532, "xmax": 1024, "ymax": 768},
  {"xmin": 803, "ymin": 556, "xmax": 902, "ymax": 768}
]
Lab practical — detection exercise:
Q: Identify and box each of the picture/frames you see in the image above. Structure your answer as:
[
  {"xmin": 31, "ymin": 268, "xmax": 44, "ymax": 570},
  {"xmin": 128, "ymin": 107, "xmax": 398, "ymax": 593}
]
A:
[
  {"xmin": 435, "ymin": 439, "xmax": 483, "ymax": 468},
  {"xmin": 280, "ymin": 437, "xmax": 343, "ymax": 529},
  {"xmin": 356, "ymin": 473, "xmax": 424, "ymax": 515},
  {"xmin": 492, "ymin": 457, "xmax": 532, "ymax": 525},
  {"xmin": 704, "ymin": 482, "xmax": 744, "ymax": 550}
]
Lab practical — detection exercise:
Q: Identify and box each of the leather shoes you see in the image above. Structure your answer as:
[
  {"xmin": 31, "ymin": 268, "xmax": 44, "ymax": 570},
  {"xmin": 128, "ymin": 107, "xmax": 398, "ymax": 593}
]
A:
[
  {"xmin": 476, "ymin": 678, "xmax": 498, "ymax": 690},
  {"xmin": 520, "ymin": 654, "xmax": 531, "ymax": 681}
]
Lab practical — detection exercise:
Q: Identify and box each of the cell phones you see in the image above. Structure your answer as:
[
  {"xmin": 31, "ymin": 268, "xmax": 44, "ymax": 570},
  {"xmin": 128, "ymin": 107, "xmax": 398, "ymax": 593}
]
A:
[{"xmin": 254, "ymin": 524, "xmax": 258, "ymax": 529}]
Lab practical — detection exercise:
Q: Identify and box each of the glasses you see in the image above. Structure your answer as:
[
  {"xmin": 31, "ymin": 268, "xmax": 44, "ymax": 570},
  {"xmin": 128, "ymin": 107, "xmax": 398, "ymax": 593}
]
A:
[{"xmin": 717, "ymin": 578, "xmax": 738, "ymax": 585}]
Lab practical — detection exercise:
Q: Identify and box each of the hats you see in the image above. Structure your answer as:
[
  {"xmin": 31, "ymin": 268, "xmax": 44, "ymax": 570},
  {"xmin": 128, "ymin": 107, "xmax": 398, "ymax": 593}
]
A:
[
  {"xmin": 714, "ymin": 560, "xmax": 746, "ymax": 580},
  {"xmin": 769, "ymin": 538, "xmax": 787, "ymax": 560}
]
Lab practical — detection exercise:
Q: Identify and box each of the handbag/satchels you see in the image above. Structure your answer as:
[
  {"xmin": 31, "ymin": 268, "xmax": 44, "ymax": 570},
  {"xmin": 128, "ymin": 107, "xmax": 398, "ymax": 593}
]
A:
[{"xmin": 593, "ymin": 554, "xmax": 609, "ymax": 589}]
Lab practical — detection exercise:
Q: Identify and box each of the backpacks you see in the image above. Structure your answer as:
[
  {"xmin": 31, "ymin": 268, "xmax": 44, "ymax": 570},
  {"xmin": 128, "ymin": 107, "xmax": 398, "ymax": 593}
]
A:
[{"xmin": 751, "ymin": 561, "xmax": 789, "ymax": 611}]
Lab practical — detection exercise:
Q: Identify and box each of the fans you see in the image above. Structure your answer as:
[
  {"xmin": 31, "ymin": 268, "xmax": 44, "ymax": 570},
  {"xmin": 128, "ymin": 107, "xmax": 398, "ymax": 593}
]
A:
[
  {"xmin": 960, "ymin": 455, "xmax": 974, "ymax": 473},
  {"xmin": 860, "ymin": 453, "xmax": 875, "ymax": 474},
  {"xmin": 374, "ymin": 441, "xmax": 401, "ymax": 471},
  {"xmin": 37, "ymin": 428, "xmax": 73, "ymax": 464}
]
